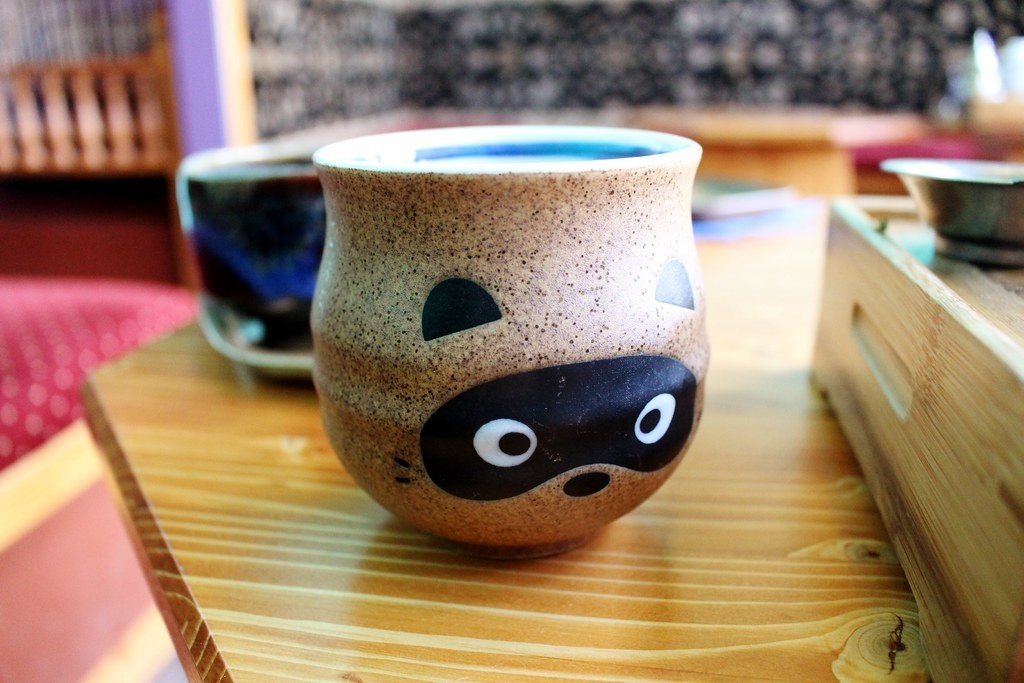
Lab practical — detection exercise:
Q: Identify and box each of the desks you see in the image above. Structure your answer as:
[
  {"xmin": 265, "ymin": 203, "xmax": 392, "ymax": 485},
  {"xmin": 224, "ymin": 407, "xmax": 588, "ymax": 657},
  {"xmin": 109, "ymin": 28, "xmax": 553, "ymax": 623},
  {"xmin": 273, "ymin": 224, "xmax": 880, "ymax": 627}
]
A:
[{"xmin": 76, "ymin": 193, "xmax": 1022, "ymax": 683}]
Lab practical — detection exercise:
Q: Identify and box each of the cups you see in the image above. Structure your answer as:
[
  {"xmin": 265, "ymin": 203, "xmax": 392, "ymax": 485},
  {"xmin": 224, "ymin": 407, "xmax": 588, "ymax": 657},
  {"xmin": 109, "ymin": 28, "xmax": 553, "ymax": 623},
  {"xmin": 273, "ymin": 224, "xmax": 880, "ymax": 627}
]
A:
[
  {"xmin": 175, "ymin": 144, "xmax": 327, "ymax": 380},
  {"xmin": 311, "ymin": 124, "xmax": 711, "ymax": 560}
]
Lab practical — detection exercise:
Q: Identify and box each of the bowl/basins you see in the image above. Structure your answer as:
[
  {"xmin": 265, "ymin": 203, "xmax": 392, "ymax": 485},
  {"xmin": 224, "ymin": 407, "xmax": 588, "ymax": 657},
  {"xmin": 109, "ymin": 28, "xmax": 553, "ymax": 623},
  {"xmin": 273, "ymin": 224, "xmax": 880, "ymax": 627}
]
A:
[{"xmin": 882, "ymin": 158, "xmax": 1024, "ymax": 266}]
